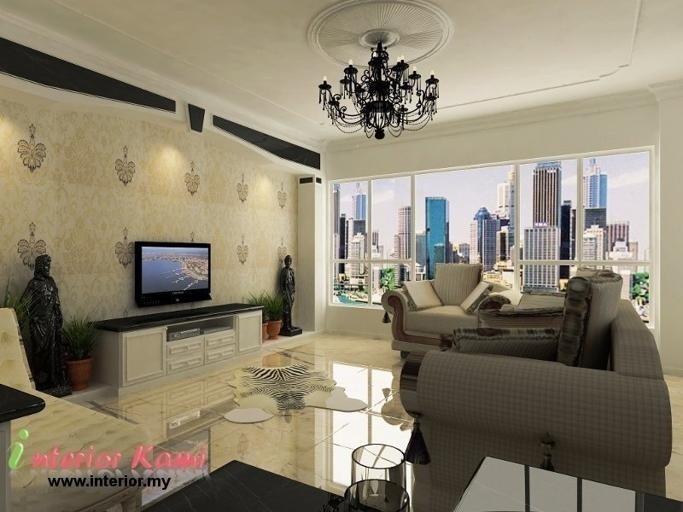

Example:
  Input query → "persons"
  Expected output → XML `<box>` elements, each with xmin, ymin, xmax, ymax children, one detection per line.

<box><xmin>14</xmin><ymin>254</ymin><xmax>73</xmax><ymax>397</ymax></box>
<box><xmin>278</xmin><ymin>254</ymin><xmax>302</xmax><ymax>337</ymax></box>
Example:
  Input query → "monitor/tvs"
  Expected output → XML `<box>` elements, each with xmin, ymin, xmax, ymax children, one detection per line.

<box><xmin>135</xmin><ymin>240</ymin><xmax>212</xmax><ymax>307</ymax></box>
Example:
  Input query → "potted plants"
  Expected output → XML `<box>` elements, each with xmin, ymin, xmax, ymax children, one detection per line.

<box><xmin>60</xmin><ymin>317</ymin><xmax>94</xmax><ymax>392</ymax></box>
<box><xmin>243</xmin><ymin>289</ymin><xmax>284</xmax><ymax>342</ymax></box>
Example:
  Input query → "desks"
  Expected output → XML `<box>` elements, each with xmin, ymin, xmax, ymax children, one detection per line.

<box><xmin>142</xmin><ymin>452</ymin><xmax>682</xmax><ymax>510</ymax></box>
<box><xmin>0</xmin><ymin>382</ymin><xmax>46</xmax><ymax>511</ymax></box>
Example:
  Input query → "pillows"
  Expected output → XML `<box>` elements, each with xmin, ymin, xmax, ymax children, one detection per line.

<box><xmin>446</xmin><ymin>326</ymin><xmax>559</xmax><ymax>361</ymax></box>
<box><xmin>399</xmin><ymin>260</ymin><xmax>492</xmax><ymax>315</ymax></box>
<box><xmin>557</xmin><ymin>268</ymin><xmax>624</xmax><ymax>368</ymax></box>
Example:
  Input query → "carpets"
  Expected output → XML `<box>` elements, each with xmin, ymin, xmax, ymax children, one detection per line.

<box><xmin>221</xmin><ymin>361</ymin><xmax>369</xmax><ymax>426</ymax></box>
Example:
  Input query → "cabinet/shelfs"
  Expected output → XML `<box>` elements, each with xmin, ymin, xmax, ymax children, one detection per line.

<box><xmin>165</xmin><ymin>335</ymin><xmax>204</xmax><ymax>373</ymax></box>
<box><xmin>204</xmin><ymin>331</ymin><xmax>237</xmax><ymax>364</ymax></box>
<box><xmin>233</xmin><ymin>311</ymin><xmax>262</xmax><ymax>354</ymax></box>
<box><xmin>90</xmin><ymin>328</ymin><xmax>164</xmax><ymax>386</ymax></box>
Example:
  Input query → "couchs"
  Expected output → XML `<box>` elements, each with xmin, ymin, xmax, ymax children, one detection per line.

<box><xmin>0</xmin><ymin>305</ymin><xmax>150</xmax><ymax>510</ymax></box>
<box><xmin>398</xmin><ymin>299</ymin><xmax>672</xmax><ymax>502</ymax></box>
<box><xmin>379</xmin><ymin>279</ymin><xmax>565</xmax><ymax>352</ymax></box>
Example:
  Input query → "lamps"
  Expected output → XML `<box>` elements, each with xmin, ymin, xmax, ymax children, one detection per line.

<box><xmin>303</xmin><ymin>2</ymin><xmax>452</xmax><ymax>141</ymax></box>
